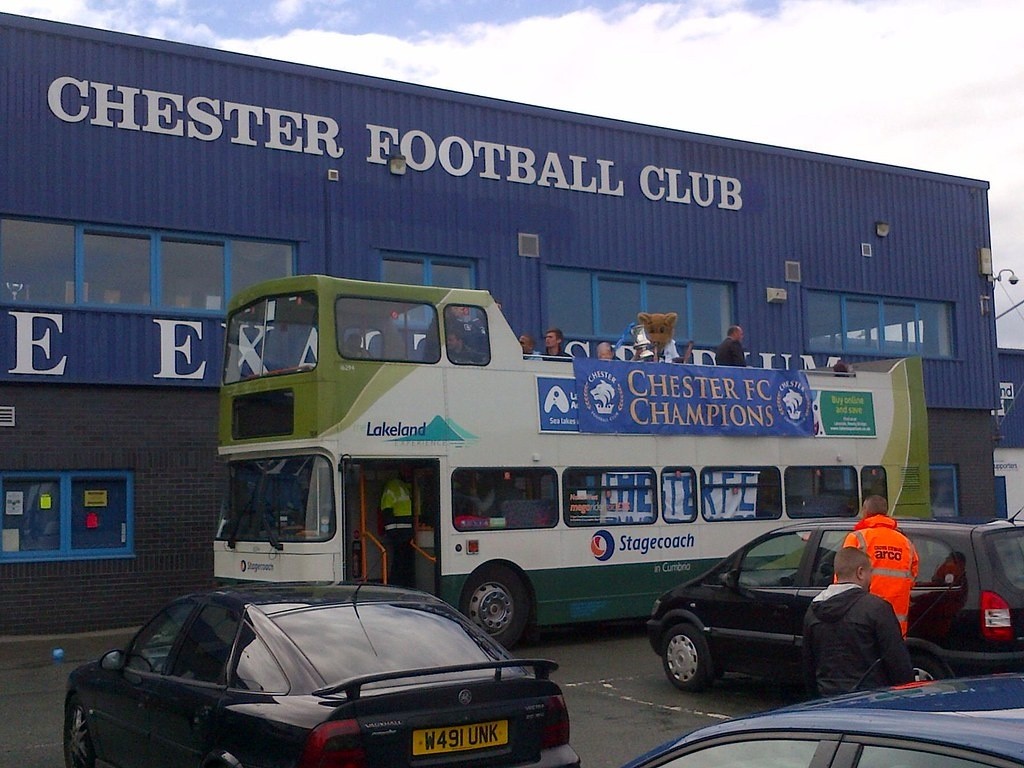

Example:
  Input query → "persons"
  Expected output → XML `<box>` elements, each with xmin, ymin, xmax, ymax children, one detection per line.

<box><xmin>425</xmin><ymin>301</ymin><xmax>504</xmax><ymax>365</ymax></box>
<box><xmin>381</xmin><ymin>464</ymin><xmax>422</xmax><ymax>591</ymax></box>
<box><xmin>342</xmin><ymin>332</ymin><xmax>371</xmax><ymax>358</ymax></box>
<box><xmin>834</xmin><ymin>495</ymin><xmax>918</xmax><ymax>640</ymax></box>
<box><xmin>453</xmin><ymin>470</ymin><xmax>496</xmax><ymax>518</ymax></box>
<box><xmin>714</xmin><ymin>326</ymin><xmax>745</xmax><ymax>367</ymax></box>
<box><xmin>596</xmin><ymin>341</ymin><xmax>621</xmax><ymax>360</ymax></box>
<box><xmin>518</xmin><ymin>335</ymin><xmax>542</xmax><ymax>361</ymax></box>
<box><xmin>801</xmin><ymin>546</ymin><xmax>916</xmax><ymax>699</ymax></box>
<box><xmin>542</xmin><ymin>329</ymin><xmax>572</xmax><ymax>361</ymax></box>
<box><xmin>834</xmin><ymin>364</ymin><xmax>850</xmax><ymax>376</ymax></box>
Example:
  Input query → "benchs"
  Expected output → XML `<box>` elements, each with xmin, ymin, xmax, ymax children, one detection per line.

<box><xmin>761</xmin><ymin>489</ymin><xmax>866</xmax><ymax>520</ymax></box>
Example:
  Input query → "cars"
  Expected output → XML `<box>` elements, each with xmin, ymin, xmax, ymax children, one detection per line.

<box><xmin>62</xmin><ymin>585</ymin><xmax>582</xmax><ymax>768</ymax></box>
<box><xmin>622</xmin><ymin>669</ymin><xmax>1024</xmax><ymax>768</ymax></box>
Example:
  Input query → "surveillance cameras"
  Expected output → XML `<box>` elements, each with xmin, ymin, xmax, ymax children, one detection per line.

<box><xmin>1009</xmin><ymin>276</ymin><xmax>1019</xmax><ymax>284</ymax></box>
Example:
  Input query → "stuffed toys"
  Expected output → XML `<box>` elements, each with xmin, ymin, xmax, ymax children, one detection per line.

<box><xmin>636</xmin><ymin>311</ymin><xmax>679</xmax><ymax>363</ymax></box>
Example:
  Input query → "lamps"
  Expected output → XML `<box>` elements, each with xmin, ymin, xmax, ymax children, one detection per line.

<box><xmin>875</xmin><ymin>220</ymin><xmax>891</xmax><ymax>238</ymax></box>
<box><xmin>388</xmin><ymin>153</ymin><xmax>409</xmax><ymax>175</ymax></box>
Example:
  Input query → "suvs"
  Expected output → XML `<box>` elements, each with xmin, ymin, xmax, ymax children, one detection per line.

<box><xmin>643</xmin><ymin>515</ymin><xmax>1024</xmax><ymax>696</ymax></box>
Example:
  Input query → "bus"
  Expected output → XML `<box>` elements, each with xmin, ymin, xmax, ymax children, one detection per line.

<box><xmin>210</xmin><ymin>274</ymin><xmax>934</xmax><ymax>650</ymax></box>
